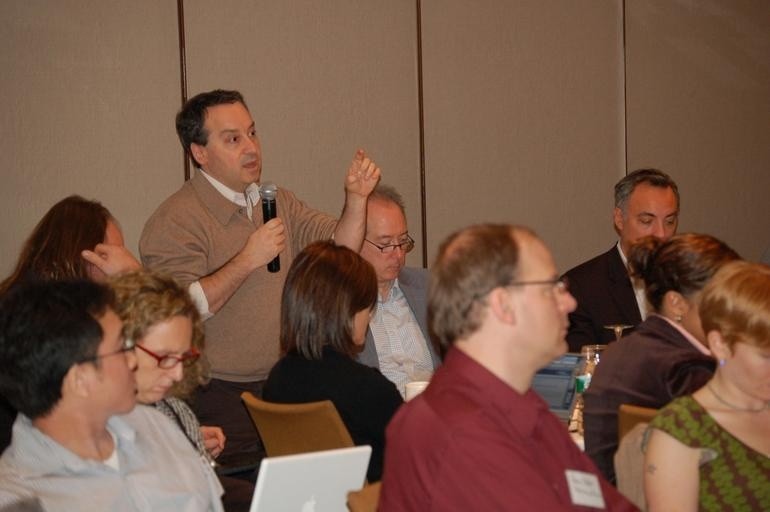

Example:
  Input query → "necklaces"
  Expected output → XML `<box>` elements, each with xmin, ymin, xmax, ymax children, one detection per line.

<box><xmin>703</xmin><ymin>381</ymin><xmax>768</xmax><ymax>413</ymax></box>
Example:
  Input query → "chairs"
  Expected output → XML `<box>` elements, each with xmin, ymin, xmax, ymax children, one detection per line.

<box><xmin>617</xmin><ymin>403</ymin><xmax>659</xmax><ymax>448</ymax></box>
<box><xmin>346</xmin><ymin>479</ymin><xmax>383</xmax><ymax>512</ymax></box>
<box><xmin>241</xmin><ymin>391</ymin><xmax>369</xmax><ymax>491</ymax></box>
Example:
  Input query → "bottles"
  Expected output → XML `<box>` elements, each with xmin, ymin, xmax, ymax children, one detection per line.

<box><xmin>575</xmin><ymin>345</ymin><xmax>597</xmax><ymax>436</ymax></box>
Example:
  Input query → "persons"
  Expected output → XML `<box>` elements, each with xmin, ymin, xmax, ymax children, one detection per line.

<box><xmin>582</xmin><ymin>232</ymin><xmax>746</xmax><ymax>489</ymax></box>
<box><xmin>644</xmin><ymin>260</ymin><xmax>770</xmax><ymax>511</ymax></box>
<box><xmin>106</xmin><ymin>267</ymin><xmax>216</xmax><ymax>469</ymax></box>
<box><xmin>1</xmin><ymin>194</ymin><xmax>227</xmax><ymax>461</ymax></box>
<box><xmin>264</xmin><ymin>237</ymin><xmax>407</xmax><ymax>483</ymax></box>
<box><xmin>0</xmin><ymin>277</ymin><xmax>228</xmax><ymax>510</ymax></box>
<box><xmin>381</xmin><ymin>222</ymin><xmax>643</xmax><ymax>512</ymax></box>
<box><xmin>355</xmin><ymin>185</ymin><xmax>442</xmax><ymax>402</ymax></box>
<box><xmin>137</xmin><ymin>86</ymin><xmax>382</xmax><ymax>511</ymax></box>
<box><xmin>557</xmin><ymin>167</ymin><xmax>681</xmax><ymax>353</ymax></box>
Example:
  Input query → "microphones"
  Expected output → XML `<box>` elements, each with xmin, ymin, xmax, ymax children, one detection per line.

<box><xmin>258</xmin><ymin>180</ymin><xmax>281</xmax><ymax>272</ymax></box>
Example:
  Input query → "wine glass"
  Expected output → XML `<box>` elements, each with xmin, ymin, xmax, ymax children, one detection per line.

<box><xmin>602</xmin><ymin>322</ymin><xmax>634</xmax><ymax>341</ymax></box>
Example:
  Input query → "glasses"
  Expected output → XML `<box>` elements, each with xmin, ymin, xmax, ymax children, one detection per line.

<box><xmin>502</xmin><ymin>276</ymin><xmax>569</xmax><ymax>294</ymax></box>
<box><xmin>364</xmin><ymin>236</ymin><xmax>415</xmax><ymax>254</ymax></box>
<box><xmin>79</xmin><ymin>340</ymin><xmax>136</xmax><ymax>364</ymax></box>
<box><xmin>134</xmin><ymin>344</ymin><xmax>201</xmax><ymax>370</ymax></box>
